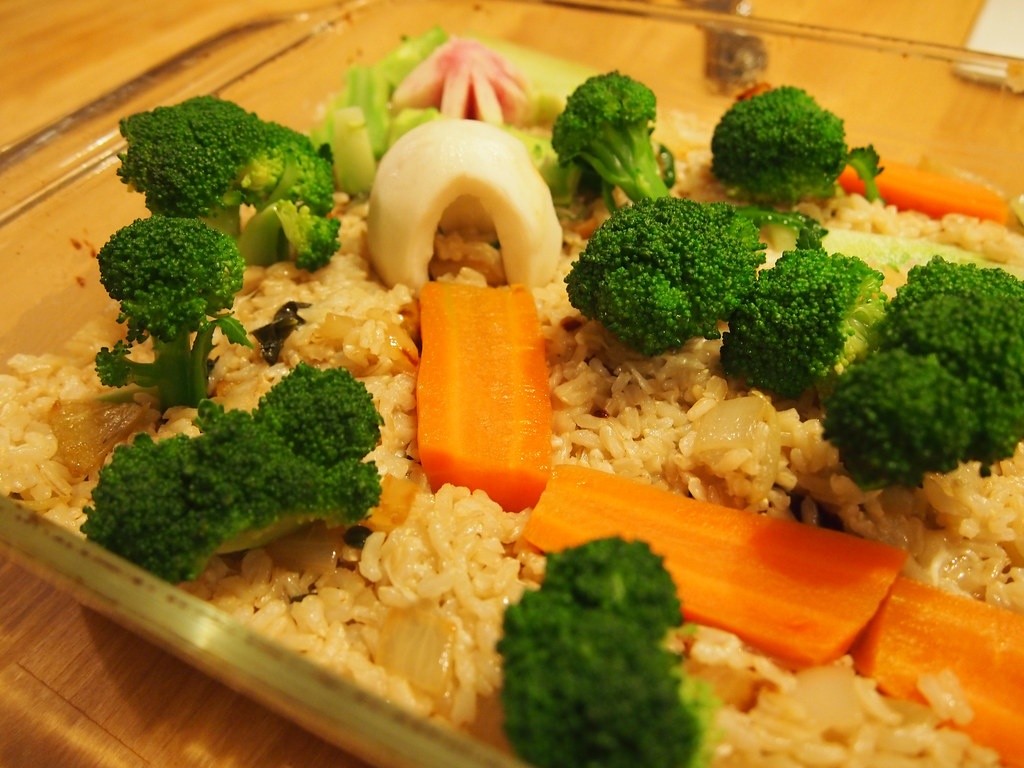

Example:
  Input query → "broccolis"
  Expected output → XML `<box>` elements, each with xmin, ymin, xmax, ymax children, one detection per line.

<box><xmin>78</xmin><ymin>22</ymin><xmax>1024</xmax><ymax>768</ymax></box>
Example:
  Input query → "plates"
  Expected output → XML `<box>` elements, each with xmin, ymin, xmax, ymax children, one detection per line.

<box><xmin>0</xmin><ymin>0</ymin><xmax>1024</xmax><ymax>768</ymax></box>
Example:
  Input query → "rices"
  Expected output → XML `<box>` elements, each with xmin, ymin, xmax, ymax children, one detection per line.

<box><xmin>1</xmin><ymin>156</ymin><xmax>1024</xmax><ymax>768</ymax></box>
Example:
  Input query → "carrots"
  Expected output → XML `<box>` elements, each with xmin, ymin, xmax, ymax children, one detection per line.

<box><xmin>519</xmin><ymin>465</ymin><xmax>911</xmax><ymax>668</ymax></box>
<box><xmin>415</xmin><ymin>281</ymin><xmax>553</xmax><ymax>511</ymax></box>
<box><xmin>852</xmin><ymin>578</ymin><xmax>1024</xmax><ymax>768</ymax></box>
<box><xmin>836</xmin><ymin>156</ymin><xmax>1008</xmax><ymax>226</ymax></box>
